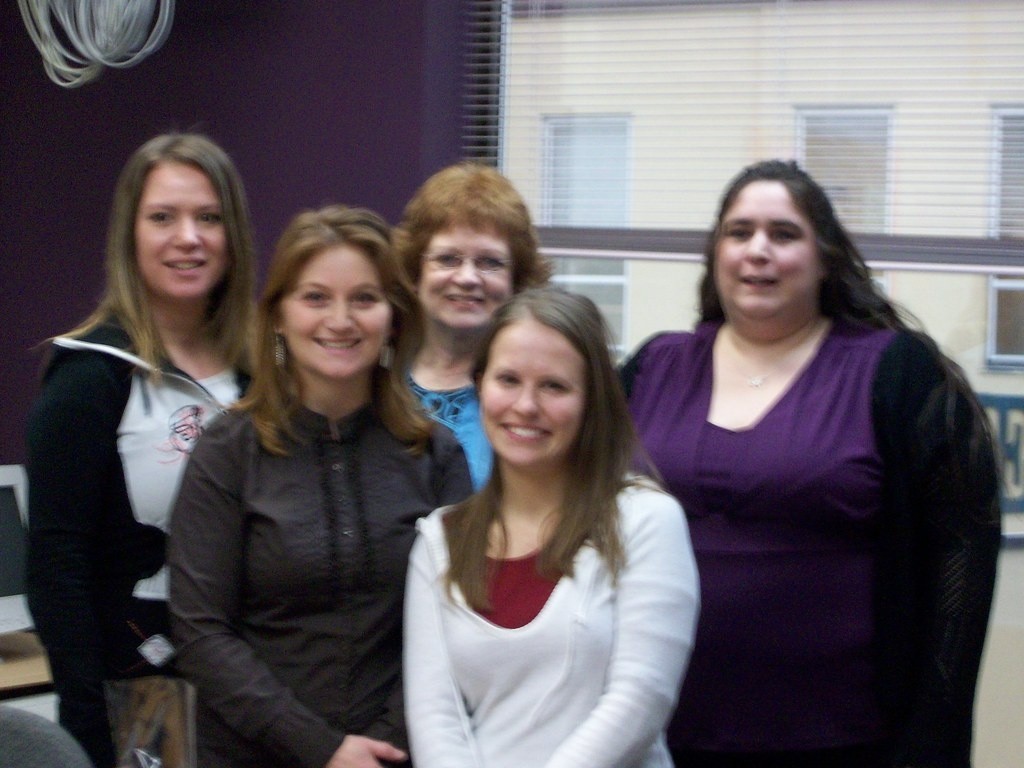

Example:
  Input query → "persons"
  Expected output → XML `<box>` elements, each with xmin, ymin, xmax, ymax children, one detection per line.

<box><xmin>399</xmin><ymin>279</ymin><xmax>702</xmax><ymax>768</ymax></box>
<box><xmin>23</xmin><ymin>130</ymin><xmax>263</xmax><ymax>768</ymax></box>
<box><xmin>165</xmin><ymin>203</ymin><xmax>476</xmax><ymax>768</ymax></box>
<box><xmin>385</xmin><ymin>161</ymin><xmax>559</xmax><ymax>490</ymax></box>
<box><xmin>613</xmin><ymin>157</ymin><xmax>1004</xmax><ymax>768</ymax></box>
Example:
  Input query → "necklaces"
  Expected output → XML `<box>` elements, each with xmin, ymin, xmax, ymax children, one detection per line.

<box><xmin>720</xmin><ymin>323</ymin><xmax>823</xmax><ymax>387</ymax></box>
